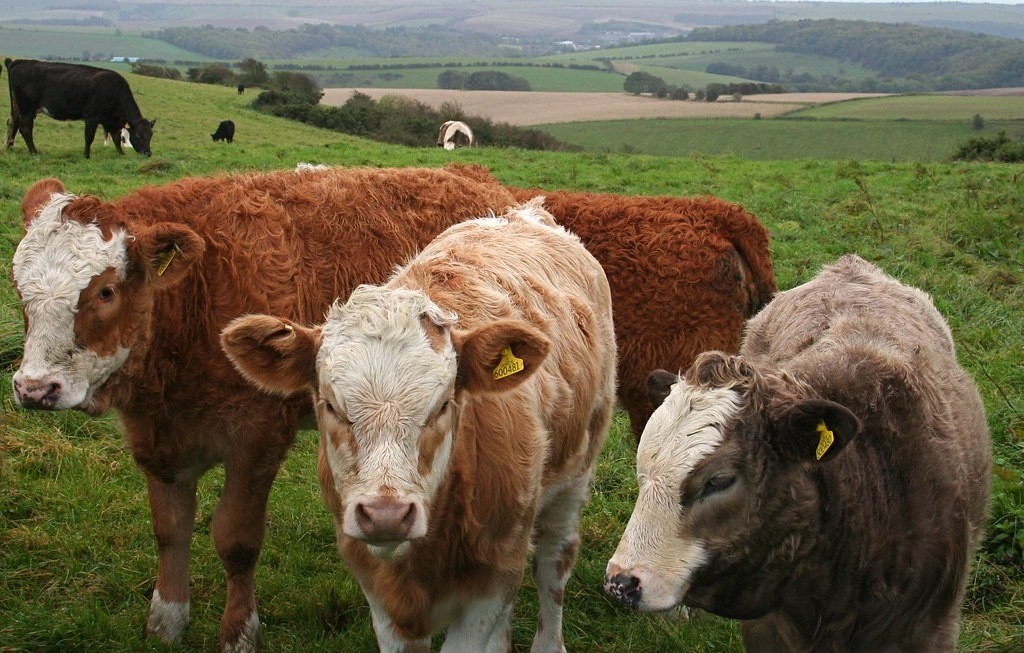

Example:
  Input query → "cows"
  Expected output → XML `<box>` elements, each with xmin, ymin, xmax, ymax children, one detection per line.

<box><xmin>603</xmin><ymin>255</ymin><xmax>990</xmax><ymax>653</ymax></box>
<box><xmin>0</xmin><ymin>58</ymin><xmax>157</xmax><ymax>158</ymax></box>
<box><xmin>219</xmin><ymin>195</ymin><xmax>616</xmax><ymax>653</ymax></box>
<box><xmin>237</xmin><ymin>84</ymin><xmax>245</xmax><ymax>95</ymax></box>
<box><xmin>8</xmin><ymin>159</ymin><xmax>772</xmax><ymax>653</ymax></box>
<box><xmin>437</xmin><ymin>121</ymin><xmax>475</xmax><ymax>151</ymax></box>
<box><xmin>210</xmin><ymin>119</ymin><xmax>236</xmax><ymax>144</ymax></box>
